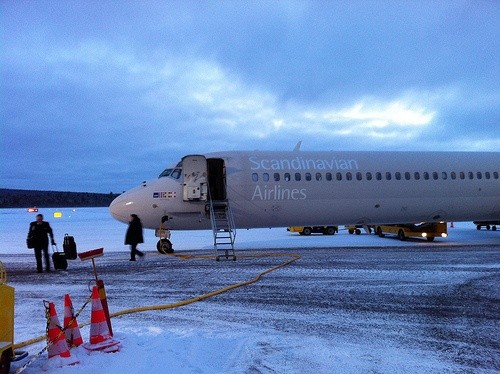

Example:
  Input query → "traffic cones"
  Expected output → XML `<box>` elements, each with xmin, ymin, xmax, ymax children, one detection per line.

<box><xmin>79</xmin><ymin>285</ymin><xmax>121</xmax><ymax>351</ymax></box>
<box><xmin>61</xmin><ymin>294</ymin><xmax>85</xmax><ymax>350</ymax></box>
<box><xmin>41</xmin><ymin>302</ymin><xmax>79</xmax><ymax>368</ymax></box>
<box><xmin>95</xmin><ymin>280</ymin><xmax>116</xmax><ymax>339</ymax></box>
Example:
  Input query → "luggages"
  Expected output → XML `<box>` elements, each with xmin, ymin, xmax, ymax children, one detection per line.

<box><xmin>51</xmin><ymin>243</ymin><xmax>68</xmax><ymax>270</ymax></box>
<box><xmin>63</xmin><ymin>233</ymin><xmax>77</xmax><ymax>260</ymax></box>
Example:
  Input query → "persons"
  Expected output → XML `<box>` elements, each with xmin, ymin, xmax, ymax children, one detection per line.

<box><xmin>125</xmin><ymin>214</ymin><xmax>144</xmax><ymax>261</ymax></box>
<box><xmin>29</xmin><ymin>214</ymin><xmax>56</xmax><ymax>274</ymax></box>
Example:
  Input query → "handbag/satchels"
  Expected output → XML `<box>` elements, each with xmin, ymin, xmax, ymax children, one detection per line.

<box><xmin>64</xmin><ymin>236</ymin><xmax>74</xmax><ymax>244</ymax></box>
<box><xmin>27</xmin><ymin>238</ymin><xmax>34</xmax><ymax>249</ymax></box>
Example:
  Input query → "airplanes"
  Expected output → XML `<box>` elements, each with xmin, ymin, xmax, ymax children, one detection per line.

<box><xmin>108</xmin><ymin>140</ymin><xmax>500</xmax><ymax>263</ymax></box>
